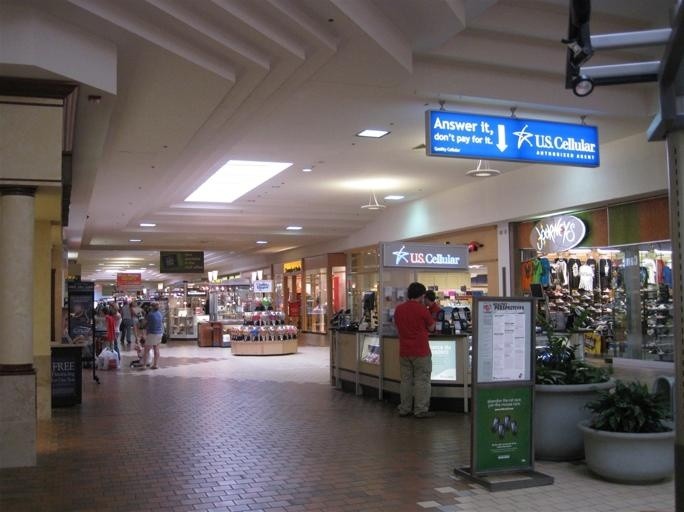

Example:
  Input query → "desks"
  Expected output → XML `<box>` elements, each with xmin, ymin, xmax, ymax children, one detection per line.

<box><xmin>328</xmin><ymin>327</ymin><xmax>472</xmax><ymax>414</ymax></box>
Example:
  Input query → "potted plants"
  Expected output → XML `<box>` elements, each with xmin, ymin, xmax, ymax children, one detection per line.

<box><xmin>532</xmin><ymin>302</ymin><xmax>613</xmax><ymax>461</ymax></box>
<box><xmin>578</xmin><ymin>381</ymin><xmax>674</xmax><ymax>484</ymax></box>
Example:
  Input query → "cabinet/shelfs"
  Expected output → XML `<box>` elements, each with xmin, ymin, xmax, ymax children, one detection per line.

<box><xmin>169</xmin><ymin>286</ymin><xmax>191</xmax><ymax>339</ymax></box>
<box><xmin>209</xmin><ymin>277</ymin><xmax>253</xmax><ymax>347</ymax></box>
<box><xmin>533</xmin><ymin>250</ymin><xmax>613</xmax><ymax>356</ymax></box>
<box><xmin>185</xmin><ymin>281</ymin><xmax>209</xmax><ymax>338</ymax></box>
<box><xmin>151</xmin><ymin>293</ymin><xmax>168</xmax><ymax>340</ymax></box>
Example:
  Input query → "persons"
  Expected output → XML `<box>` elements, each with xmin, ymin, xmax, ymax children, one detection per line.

<box><xmin>423</xmin><ymin>289</ymin><xmax>442</xmax><ymax>321</ymax></box>
<box><xmin>140</xmin><ymin>337</ymin><xmax>152</xmax><ymax>367</ymax></box>
<box><xmin>102</xmin><ymin>302</ymin><xmax>106</xmax><ymax>308</ymax></box>
<box><xmin>394</xmin><ymin>282</ymin><xmax>436</xmax><ymax>419</ymax></box>
<box><xmin>119</xmin><ymin>306</ymin><xmax>122</xmax><ymax>315</ymax></box>
<box><xmin>261</xmin><ymin>297</ymin><xmax>273</xmax><ymax>310</ymax></box>
<box><xmin>203</xmin><ymin>299</ymin><xmax>209</xmax><ymax>314</ymax></box>
<box><xmin>141</xmin><ymin>302</ymin><xmax>152</xmax><ymax>317</ymax></box>
<box><xmin>100</xmin><ymin>307</ymin><xmax>116</xmax><ymax>352</ymax></box>
<box><xmin>138</xmin><ymin>302</ymin><xmax>163</xmax><ymax>370</ymax></box>
<box><xmin>106</xmin><ymin>302</ymin><xmax>109</xmax><ymax>308</ymax></box>
<box><xmin>254</xmin><ymin>298</ymin><xmax>265</xmax><ymax>311</ymax></box>
<box><xmin>313</xmin><ymin>296</ymin><xmax>327</xmax><ymax>313</ymax></box>
<box><xmin>119</xmin><ymin>301</ymin><xmax>133</xmax><ymax>345</ymax></box>
<box><xmin>130</xmin><ymin>301</ymin><xmax>143</xmax><ymax>345</ymax></box>
<box><xmin>111</xmin><ymin>303</ymin><xmax>122</xmax><ymax>364</ymax></box>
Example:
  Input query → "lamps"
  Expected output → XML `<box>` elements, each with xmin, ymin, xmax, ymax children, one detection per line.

<box><xmin>466</xmin><ymin>161</ymin><xmax>501</xmax><ymax>178</ymax></box>
<box><xmin>184</xmin><ymin>160</ymin><xmax>293</xmax><ymax>203</ymax></box>
<box><xmin>574</xmin><ymin>68</ymin><xmax>594</xmax><ymax>97</ymax></box>
<box><xmin>361</xmin><ymin>191</ymin><xmax>386</xmax><ymax>210</ymax></box>
